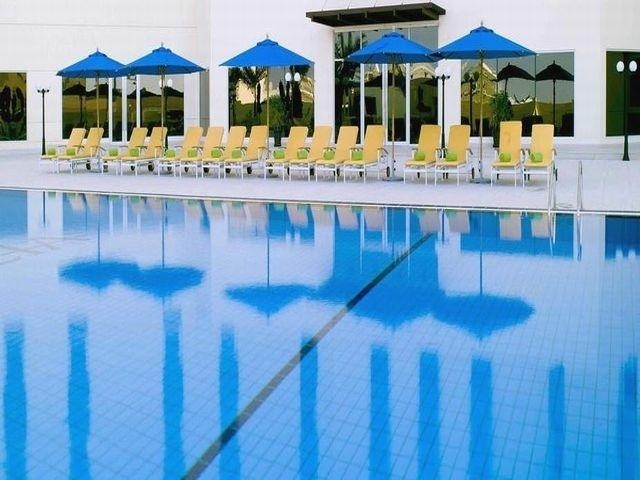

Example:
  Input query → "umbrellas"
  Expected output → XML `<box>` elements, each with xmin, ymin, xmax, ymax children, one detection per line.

<box><xmin>490</xmin><ymin>59</ymin><xmax>574</xmax><ymax>125</ymax></box>
<box><xmin>218</xmin><ymin>34</ymin><xmax>315</xmax><ymax>173</ymax></box>
<box><xmin>346</xmin><ymin>27</ymin><xmax>442</xmax><ymax>178</ymax></box>
<box><xmin>116</xmin><ymin>42</ymin><xmax>208</xmax><ymax>171</ymax></box>
<box><xmin>55</xmin><ymin>48</ymin><xmax>128</xmax><ymax>127</ymax></box>
<box><xmin>430</xmin><ymin>21</ymin><xmax>537</xmax><ymax>178</ymax></box>
<box><xmin>63</xmin><ymin>82</ymin><xmax>183</xmax><ymax>127</ymax></box>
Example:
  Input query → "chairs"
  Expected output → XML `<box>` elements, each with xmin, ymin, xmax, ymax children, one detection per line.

<box><xmin>179</xmin><ymin>127</ymin><xmax>224</xmax><ymax>179</ymax></box>
<box><xmin>315</xmin><ymin>127</ymin><xmax>359</xmax><ymax>183</ymax></box>
<box><xmin>156</xmin><ymin>127</ymin><xmax>203</xmax><ymax>178</ymax></box>
<box><xmin>55</xmin><ymin>128</ymin><xmax>106</xmax><ymax>176</ymax></box>
<box><xmin>64</xmin><ymin>192</ymin><xmax>560</xmax><ymax>245</ymax></box>
<box><xmin>120</xmin><ymin>127</ymin><xmax>168</xmax><ymax>177</ymax></box>
<box><xmin>489</xmin><ymin>121</ymin><xmax>525</xmax><ymax>188</ymax></box>
<box><xmin>221</xmin><ymin>126</ymin><xmax>269</xmax><ymax>181</ymax></box>
<box><xmin>100</xmin><ymin>128</ymin><xmax>148</xmax><ymax>177</ymax></box>
<box><xmin>41</xmin><ymin>128</ymin><xmax>87</xmax><ymax>174</ymax></box>
<box><xmin>201</xmin><ymin>126</ymin><xmax>247</xmax><ymax>180</ymax></box>
<box><xmin>523</xmin><ymin>123</ymin><xmax>558</xmax><ymax>188</ymax></box>
<box><xmin>262</xmin><ymin>126</ymin><xmax>308</xmax><ymax>181</ymax></box>
<box><xmin>401</xmin><ymin>124</ymin><xmax>442</xmax><ymax>185</ymax></box>
<box><xmin>288</xmin><ymin>126</ymin><xmax>333</xmax><ymax>181</ymax></box>
<box><xmin>342</xmin><ymin>125</ymin><xmax>396</xmax><ymax>184</ymax></box>
<box><xmin>434</xmin><ymin>124</ymin><xmax>475</xmax><ymax>187</ymax></box>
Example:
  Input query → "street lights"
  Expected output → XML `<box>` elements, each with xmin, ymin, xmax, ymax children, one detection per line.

<box><xmin>616</xmin><ymin>60</ymin><xmax>639</xmax><ymax>160</ymax></box>
<box><xmin>285</xmin><ymin>71</ymin><xmax>303</xmax><ymax>127</ymax></box>
<box><xmin>37</xmin><ymin>88</ymin><xmax>50</xmax><ymax>156</ymax></box>
<box><xmin>157</xmin><ymin>79</ymin><xmax>174</xmax><ymax>129</ymax></box>
<box><xmin>463</xmin><ymin>72</ymin><xmax>479</xmax><ymax>132</ymax></box>
